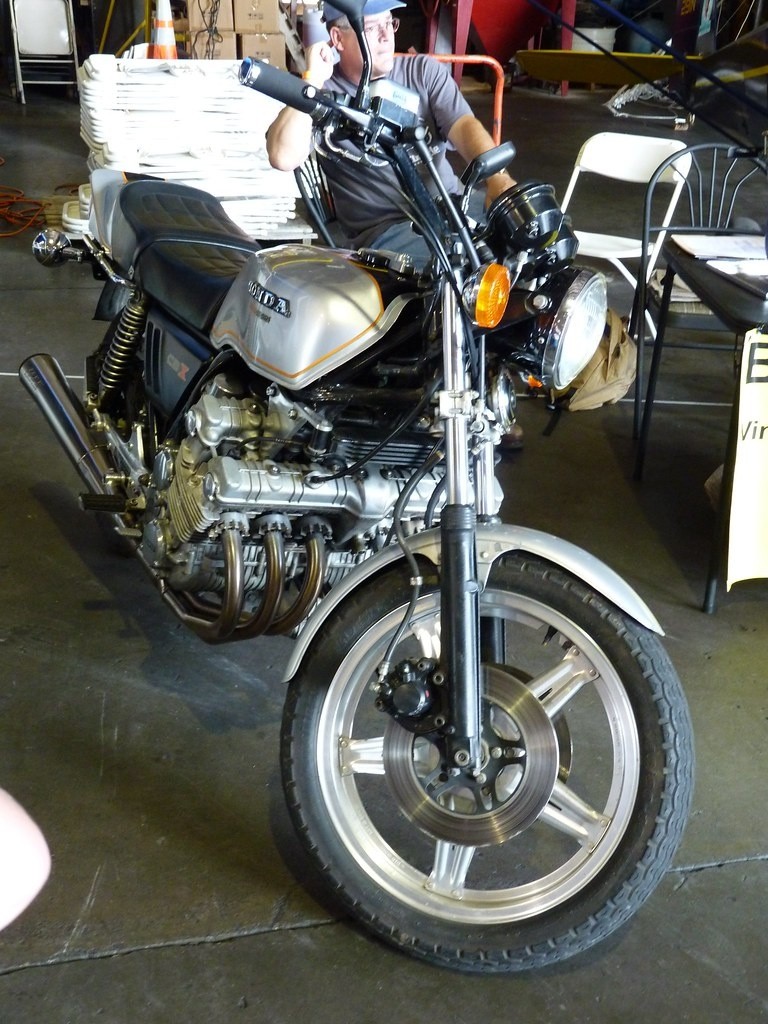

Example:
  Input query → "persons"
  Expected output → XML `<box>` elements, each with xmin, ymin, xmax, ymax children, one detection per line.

<box><xmin>265</xmin><ymin>0</ymin><xmax>525</xmax><ymax>450</ymax></box>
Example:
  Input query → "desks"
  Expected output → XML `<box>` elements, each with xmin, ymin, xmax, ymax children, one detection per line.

<box><xmin>635</xmin><ymin>235</ymin><xmax>768</xmax><ymax>615</ymax></box>
<box><xmin>627</xmin><ymin>142</ymin><xmax>768</xmax><ymax>473</ymax></box>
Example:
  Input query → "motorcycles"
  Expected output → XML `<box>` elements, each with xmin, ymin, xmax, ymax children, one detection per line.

<box><xmin>18</xmin><ymin>0</ymin><xmax>696</xmax><ymax>980</ymax></box>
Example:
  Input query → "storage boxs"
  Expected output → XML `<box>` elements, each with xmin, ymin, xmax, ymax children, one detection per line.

<box><xmin>188</xmin><ymin>0</ymin><xmax>290</xmax><ymax>73</ymax></box>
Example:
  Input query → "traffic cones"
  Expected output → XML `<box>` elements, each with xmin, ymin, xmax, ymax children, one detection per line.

<box><xmin>153</xmin><ymin>0</ymin><xmax>179</xmax><ymax>59</ymax></box>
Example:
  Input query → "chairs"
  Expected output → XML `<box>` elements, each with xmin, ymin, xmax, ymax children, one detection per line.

<box><xmin>298</xmin><ymin>153</ymin><xmax>340</xmax><ymax>249</ymax></box>
<box><xmin>560</xmin><ymin>131</ymin><xmax>692</xmax><ymax>337</ymax></box>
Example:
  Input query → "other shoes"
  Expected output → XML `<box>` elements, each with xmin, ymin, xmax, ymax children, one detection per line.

<box><xmin>501</xmin><ymin>424</ymin><xmax>524</xmax><ymax>450</ymax></box>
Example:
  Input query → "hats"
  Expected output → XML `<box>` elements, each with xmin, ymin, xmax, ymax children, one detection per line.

<box><xmin>320</xmin><ymin>0</ymin><xmax>407</xmax><ymax>23</ymax></box>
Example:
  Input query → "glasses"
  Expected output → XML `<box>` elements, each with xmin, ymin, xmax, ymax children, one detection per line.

<box><xmin>337</xmin><ymin>18</ymin><xmax>400</xmax><ymax>33</ymax></box>
